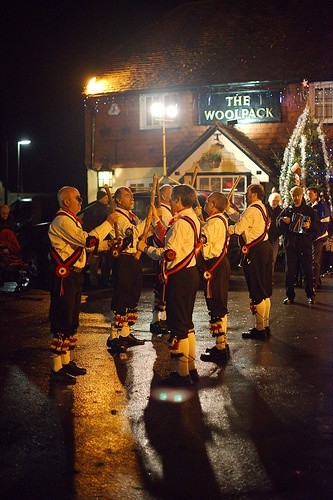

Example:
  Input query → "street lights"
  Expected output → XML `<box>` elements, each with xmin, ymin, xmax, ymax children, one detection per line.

<box><xmin>16</xmin><ymin>139</ymin><xmax>32</xmax><ymax>199</ymax></box>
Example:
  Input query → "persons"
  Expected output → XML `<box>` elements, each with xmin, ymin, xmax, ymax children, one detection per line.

<box><xmin>276</xmin><ymin>186</ymin><xmax>321</xmax><ymax>304</ymax></box>
<box><xmin>108</xmin><ymin>187</ymin><xmax>163</xmax><ymax>353</ymax></box>
<box><xmin>265</xmin><ymin>192</ymin><xmax>282</xmax><ymax>285</ymax></box>
<box><xmin>48</xmin><ymin>186</ymin><xmax>119</xmax><ymax>385</ymax></box>
<box><xmin>149</xmin><ymin>184</ymin><xmax>202</xmax><ymax>334</ymax></box>
<box><xmin>136</xmin><ymin>184</ymin><xmax>200</xmax><ymax>386</ymax></box>
<box><xmin>82</xmin><ymin>190</ymin><xmax>112</xmax><ymax>292</ymax></box>
<box><xmin>224</xmin><ymin>184</ymin><xmax>272</xmax><ymax>340</ymax></box>
<box><xmin>200</xmin><ymin>191</ymin><xmax>231</xmax><ymax>364</ymax></box>
<box><xmin>0</xmin><ymin>205</ymin><xmax>16</xmax><ymax>233</ymax></box>
<box><xmin>294</xmin><ymin>187</ymin><xmax>333</xmax><ymax>292</ymax></box>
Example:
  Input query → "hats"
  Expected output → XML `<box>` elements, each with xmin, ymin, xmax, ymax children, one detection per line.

<box><xmin>97</xmin><ymin>191</ymin><xmax>107</xmax><ymax>201</ymax></box>
<box><xmin>289</xmin><ymin>187</ymin><xmax>303</xmax><ymax>196</ymax></box>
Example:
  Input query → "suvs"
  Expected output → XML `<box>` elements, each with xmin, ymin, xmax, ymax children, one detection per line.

<box><xmin>76</xmin><ymin>191</ymin><xmax>247</xmax><ymax>274</ymax></box>
<box><xmin>4</xmin><ymin>197</ymin><xmax>58</xmax><ymax>279</ymax></box>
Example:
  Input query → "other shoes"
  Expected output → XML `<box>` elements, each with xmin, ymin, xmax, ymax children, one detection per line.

<box><xmin>242</xmin><ymin>328</ymin><xmax>266</xmax><ymax>340</ymax></box>
<box><xmin>106</xmin><ymin>337</ymin><xmax>128</xmax><ymax>350</ymax></box>
<box><xmin>308</xmin><ymin>298</ymin><xmax>313</xmax><ymax>304</ymax></box>
<box><xmin>283</xmin><ymin>297</ymin><xmax>294</xmax><ymax>304</ymax></box>
<box><xmin>161</xmin><ymin>371</ymin><xmax>191</xmax><ymax>387</ymax></box>
<box><xmin>205</xmin><ymin>344</ymin><xmax>230</xmax><ymax>354</ymax></box>
<box><xmin>190</xmin><ymin>368</ymin><xmax>200</xmax><ymax>385</ymax></box>
<box><xmin>63</xmin><ymin>361</ymin><xmax>86</xmax><ymax>376</ymax></box>
<box><xmin>150</xmin><ymin>321</ymin><xmax>166</xmax><ymax>335</ymax></box>
<box><xmin>163</xmin><ymin>319</ymin><xmax>170</xmax><ymax>330</ymax></box>
<box><xmin>249</xmin><ymin>326</ymin><xmax>271</xmax><ymax>335</ymax></box>
<box><xmin>119</xmin><ymin>333</ymin><xmax>144</xmax><ymax>345</ymax></box>
<box><xmin>50</xmin><ymin>367</ymin><xmax>77</xmax><ymax>384</ymax></box>
<box><xmin>200</xmin><ymin>348</ymin><xmax>228</xmax><ymax>363</ymax></box>
